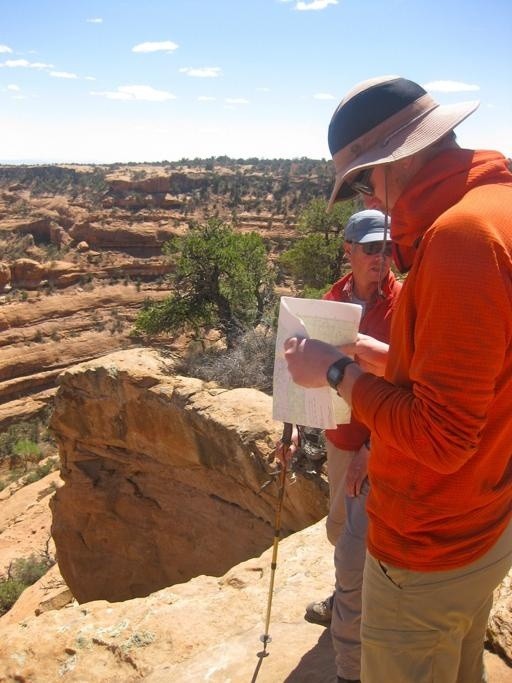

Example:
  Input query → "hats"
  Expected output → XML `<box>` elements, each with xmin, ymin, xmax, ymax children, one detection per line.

<box><xmin>345</xmin><ymin>209</ymin><xmax>392</xmax><ymax>243</ymax></box>
<box><xmin>325</xmin><ymin>77</ymin><xmax>481</xmax><ymax>214</ymax></box>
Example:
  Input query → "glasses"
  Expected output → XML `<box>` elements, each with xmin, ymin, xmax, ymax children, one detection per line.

<box><xmin>363</xmin><ymin>242</ymin><xmax>392</xmax><ymax>257</ymax></box>
<box><xmin>349</xmin><ymin>168</ymin><xmax>374</xmax><ymax>197</ymax></box>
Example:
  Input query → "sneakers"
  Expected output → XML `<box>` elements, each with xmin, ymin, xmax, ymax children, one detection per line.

<box><xmin>306</xmin><ymin>598</ymin><xmax>331</xmax><ymax>623</ymax></box>
